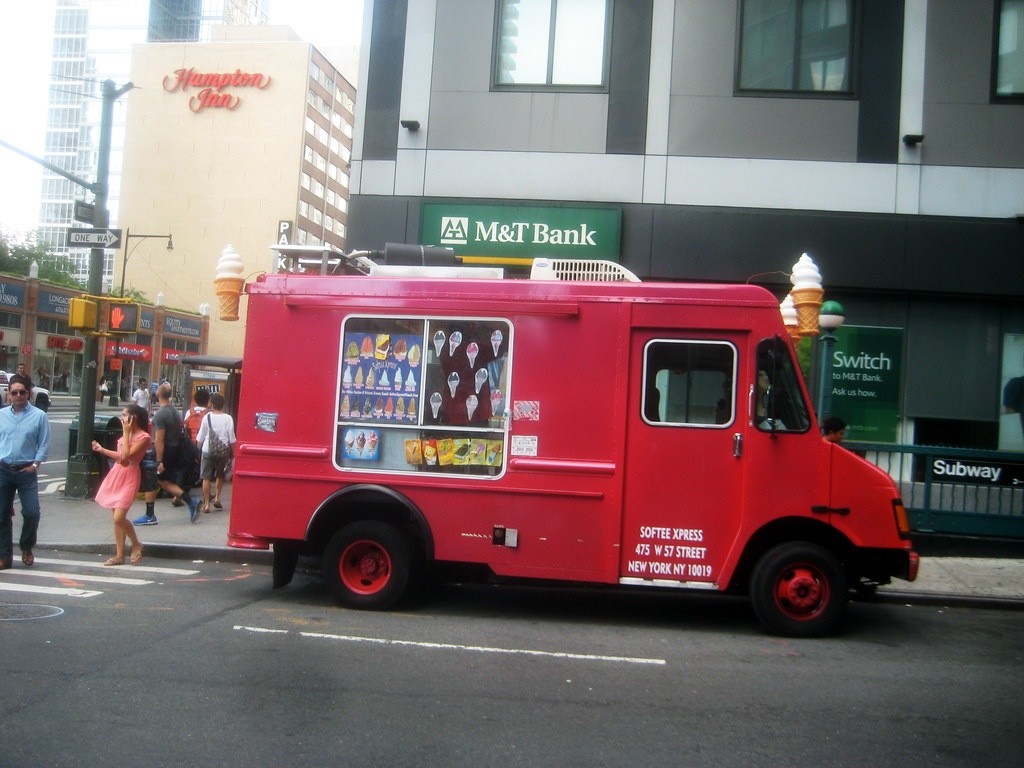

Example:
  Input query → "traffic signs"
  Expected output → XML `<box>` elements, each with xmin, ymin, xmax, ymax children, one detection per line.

<box><xmin>65</xmin><ymin>227</ymin><xmax>122</xmax><ymax>250</ymax></box>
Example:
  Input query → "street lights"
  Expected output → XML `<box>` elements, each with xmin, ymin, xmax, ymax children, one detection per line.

<box><xmin>109</xmin><ymin>227</ymin><xmax>174</xmax><ymax>406</ymax></box>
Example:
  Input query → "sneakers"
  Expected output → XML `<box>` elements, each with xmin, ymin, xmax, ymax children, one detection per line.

<box><xmin>190</xmin><ymin>497</ymin><xmax>202</xmax><ymax>523</ymax></box>
<box><xmin>132</xmin><ymin>514</ymin><xmax>158</xmax><ymax>525</ymax></box>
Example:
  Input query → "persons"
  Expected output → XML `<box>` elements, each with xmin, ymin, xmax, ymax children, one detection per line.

<box><xmin>8</xmin><ymin>364</ymin><xmax>31</xmax><ymax>406</ymax></box>
<box><xmin>0</xmin><ymin>378</ymin><xmax>50</xmax><ymax>570</ymax></box>
<box><xmin>822</xmin><ymin>417</ymin><xmax>847</xmax><ymax>444</ymax></box>
<box><xmin>107</xmin><ymin>376</ymin><xmax>170</xmax><ymax>404</ymax></box>
<box><xmin>91</xmin><ymin>405</ymin><xmax>152</xmax><ymax>566</ymax></box>
<box><xmin>438</xmin><ymin>321</ymin><xmax>508</xmax><ymax>428</ymax></box>
<box><xmin>195</xmin><ymin>393</ymin><xmax>237</xmax><ymax>513</ymax></box>
<box><xmin>132</xmin><ymin>378</ymin><xmax>152</xmax><ymax>422</ymax></box>
<box><xmin>132</xmin><ymin>383</ymin><xmax>202</xmax><ymax>526</ymax></box>
<box><xmin>98</xmin><ymin>375</ymin><xmax>109</xmax><ymax>404</ymax></box>
<box><xmin>171</xmin><ymin>388</ymin><xmax>216</xmax><ymax>506</ymax></box>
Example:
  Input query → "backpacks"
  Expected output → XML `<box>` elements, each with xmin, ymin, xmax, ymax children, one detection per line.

<box><xmin>184</xmin><ymin>406</ymin><xmax>209</xmax><ymax>449</ymax></box>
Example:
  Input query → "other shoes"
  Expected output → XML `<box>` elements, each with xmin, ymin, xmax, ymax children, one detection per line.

<box><xmin>130</xmin><ymin>542</ymin><xmax>144</xmax><ymax>563</ymax></box>
<box><xmin>174</xmin><ymin>499</ymin><xmax>185</xmax><ymax>506</ymax></box>
<box><xmin>104</xmin><ymin>556</ymin><xmax>125</xmax><ymax>565</ymax></box>
<box><xmin>204</xmin><ymin>492</ymin><xmax>216</xmax><ymax>503</ymax></box>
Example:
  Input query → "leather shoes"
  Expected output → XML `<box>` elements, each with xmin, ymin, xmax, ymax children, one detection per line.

<box><xmin>0</xmin><ymin>559</ymin><xmax>12</xmax><ymax>570</ymax></box>
<box><xmin>22</xmin><ymin>548</ymin><xmax>34</xmax><ymax>565</ymax></box>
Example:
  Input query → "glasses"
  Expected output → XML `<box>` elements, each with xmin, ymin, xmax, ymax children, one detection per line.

<box><xmin>10</xmin><ymin>390</ymin><xmax>29</xmax><ymax>396</ymax></box>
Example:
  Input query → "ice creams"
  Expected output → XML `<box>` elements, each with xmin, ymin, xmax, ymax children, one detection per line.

<box><xmin>779</xmin><ymin>292</ymin><xmax>801</xmax><ymax>353</ymax></box>
<box><xmin>343</xmin><ymin>365</ymin><xmax>416</xmax><ymax>392</ymax></box>
<box><xmin>789</xmin><ymin>253</ymin><xmax>824</xmax><ymax>335</ymax></box>
<box><xmin>213</xmin><ymin>243</ymin><xmax>245</xmax><ymax>321</ymax></box>
<box><xmin>345</xmin><ymin>429</ymin><xmax>378</xmax><ymax>450</ymax></box>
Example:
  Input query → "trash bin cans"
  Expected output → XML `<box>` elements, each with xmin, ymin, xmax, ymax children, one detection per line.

<box><xmin>68</xmin><ymin>414</ymin><xmax>123</xmax><ymax>481</ymax></box>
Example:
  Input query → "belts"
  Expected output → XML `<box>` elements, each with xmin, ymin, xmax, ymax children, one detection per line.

<box><xmin>0</xmin><ymin>461</ymin><xmax>34</xmax><ymax>471</ymax></box>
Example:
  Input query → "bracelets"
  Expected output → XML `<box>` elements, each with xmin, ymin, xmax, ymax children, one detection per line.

<box><xmin>155</xmin><ymin>461</ymin><xmax>163</xmax><ymax>470</ymax></box>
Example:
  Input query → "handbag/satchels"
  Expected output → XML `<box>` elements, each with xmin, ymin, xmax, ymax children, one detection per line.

<box><xmin>173</xmin><ymin>459</ymin><xmax>201</xmax><ymax>484</ymax></box>
<box><xmin>207</xmin><ymin>413</ymin><xmax>231</xmax><ymax>458</ymax></box>
<box><xmin>223</xmin><ymin>457</ymin><xmax>235</xmax><ymax>482</ymax></box>
<box><xmin>107</xmin><ymin>453</ymin><xmax>161</xmax><ymax>492</ymax></box>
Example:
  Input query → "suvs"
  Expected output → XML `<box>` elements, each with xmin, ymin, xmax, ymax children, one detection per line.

<box><xmin>0</xmin><ymin>371</ymin><xmax>51</xmax><ymax>413</ymax></box>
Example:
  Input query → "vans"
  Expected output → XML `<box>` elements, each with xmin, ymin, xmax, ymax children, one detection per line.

<box><xmin>227</xmin><ymin>244</ymin><xmax>920</xmax><ymax>637</ymax></box>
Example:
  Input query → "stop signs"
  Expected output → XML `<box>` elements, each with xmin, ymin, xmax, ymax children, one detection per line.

<box><xmin>107</xmin><ymin>301</ymin><xmax>141</xmax><ymax>334</ymax></box>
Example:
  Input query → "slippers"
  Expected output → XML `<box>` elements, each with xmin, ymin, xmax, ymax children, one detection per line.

<box><xmin>213</xmin><ymin>501</ymin><xmax>223</xmax><ymax>508</ymax></box>
<box><xmin>203</xmin><ymin>505</ymin><xmax>210</xmax><ymax>513</ymax></box>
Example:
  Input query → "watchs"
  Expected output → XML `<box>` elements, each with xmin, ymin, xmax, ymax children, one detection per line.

<box><xmin>33</xmin><ymin>463</ymin><xmax>39</xmax><ymax>469</ymax></box>
